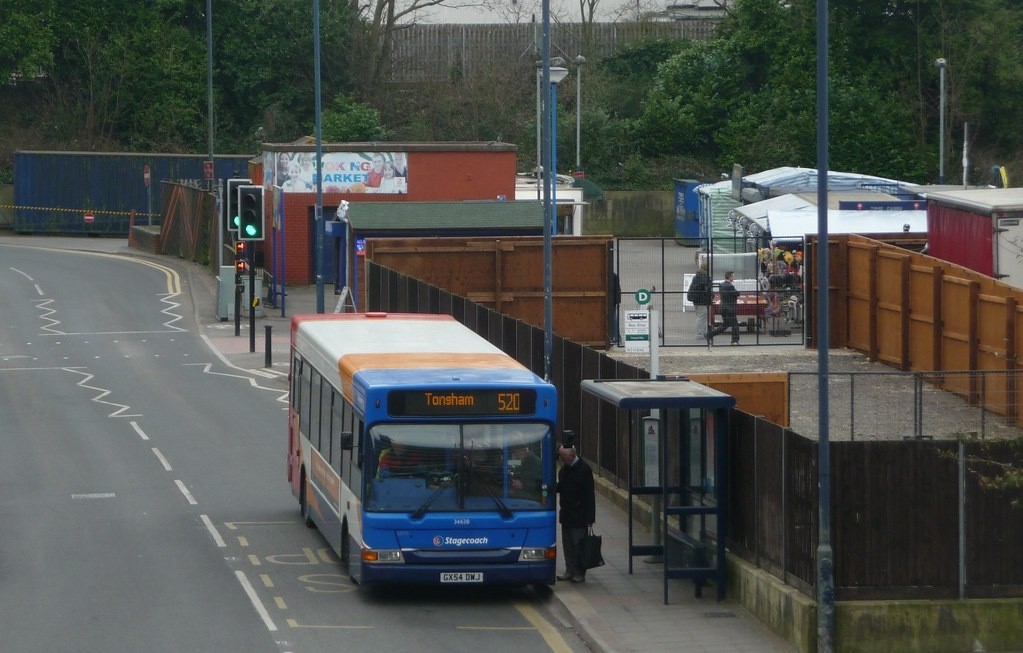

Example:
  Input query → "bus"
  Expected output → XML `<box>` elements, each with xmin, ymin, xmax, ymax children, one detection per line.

<box><xmin>287</xmin><ymin>312</ymin><xmax>561</xmax><ymax>585</ymax></box>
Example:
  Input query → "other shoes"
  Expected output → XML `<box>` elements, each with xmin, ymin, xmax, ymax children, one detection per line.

<box><xmin>572</xmin><ymin>574</ymin><xmax>585</xmax><ymax>583</ymax></box>
<box><xmin>696</xmin><ymin>334</ymin><xmax>705</xmax><ymax>340</ymax></box>
<box><xmin>706</xmin><ymin>334</ymin><xmax>712</xmax><ymax>344</ymax></box>
<box><xmin>557</xmin><ymin>572</ymin><xmax>573</xmax><ymax>580</ymax></box>
<box><xmin>731</xmin><ymin>341</ymin><xmax>737</xmax><ymax>344</ymax></box>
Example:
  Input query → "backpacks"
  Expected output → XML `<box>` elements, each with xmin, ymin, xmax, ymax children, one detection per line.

<box><xmin>687</xmin><ymin>273</ymin><xmax>704</xmax><ymax>301</ymax></box>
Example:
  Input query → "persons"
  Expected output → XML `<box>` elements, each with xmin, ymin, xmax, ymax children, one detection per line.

<box><xmin>363</xmin><ymin>152</ymin><xmax>408</xmax><ymax>193</ymax></box>
<box><xmin>509</xmin><ymin>448</ymin><xmax>542</xmax><ymax>499</ymax></box>
<box><xmin>694</xmin><ymin>264</ymin><xmax>708</xmax><ymax>340</ymax></box>
<box><xmin>706</xmin><ymin>271</ymin><xmax>740</xmax><ymax>345</ymax></box>
<box><xmin>277</xmin><ymin>153</ymin><xmax>313</xmax><ymax>191</ymax></box>
<box><xmin>379</xmin><ymin>437</ymin><xmax>421</xmax><ymax>471</ymax></box>
<box><xmin>455</xmin><ymin>438</ymin><xmax>490</xmax><ymax>478</ymax></box>
<box><xmin>557</xmin><ymin>447</ymin><xmax>596</xmax><ymax>584</ymax></box>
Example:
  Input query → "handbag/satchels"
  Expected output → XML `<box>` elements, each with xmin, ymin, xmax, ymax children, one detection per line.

<box><xmin>579</xmin><ymin>526</ymin><xmax>605</xmax><ymax>569</ymax></box>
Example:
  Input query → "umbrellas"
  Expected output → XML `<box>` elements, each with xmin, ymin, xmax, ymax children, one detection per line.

<box><xmin>573</xmin><ymin>178</ymin><xmax>605</xmax><ymax>200</ymax></box>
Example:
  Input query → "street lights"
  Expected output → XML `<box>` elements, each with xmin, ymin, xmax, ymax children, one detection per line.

<box><xmin>571</xmin><ymin>52</ymin><xmax>587</xmax><ymax>172</ymax></box>
<box><xmin>538</xmin><ymin>65</ymin><xmax>570</xmax><ymax>236</ymax></box>
<box><xmin>933</xmin><ymin>58</ymin><xmax>948</xmax><ymax>185</ymax></box>
<box><xmin>537</xmin><ymin>57</ymin><xmax>566</xmax><ymax>201</ymax></box>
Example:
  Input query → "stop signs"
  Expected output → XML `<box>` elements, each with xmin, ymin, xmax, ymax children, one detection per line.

<box><xmin>84</xmin><ymin>213</ymin><xmax>96</xmax><ymax>225</ymax></box>
<box><xmin>143</xmin><ymin>165</ymin><xmax>151</xmax><ymax>186</ymax></box>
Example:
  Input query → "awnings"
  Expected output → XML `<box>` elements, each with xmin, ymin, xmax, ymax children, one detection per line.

<box><xmin>735</xmin><ymin>194</ymin><xmax>818</xmax><ymax>232</ymax></box>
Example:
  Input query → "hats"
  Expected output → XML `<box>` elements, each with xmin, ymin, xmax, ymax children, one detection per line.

<box><xmin>699</xmin><ymin>261</ymin><xmax>708</xmax><ymax>274</ymax></box>
<box><xmin>758</xmin><ymin>248</ymin><xmax>804</xmax><ymax>323</ymax></box>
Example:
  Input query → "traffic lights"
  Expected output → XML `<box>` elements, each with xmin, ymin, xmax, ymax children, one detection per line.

<box><xmin>234</xmin><ymin>241</ymin><xmax>245</xmax><ymax>256</ymax></box>
<box><xmin>235</xmin><ymin>259</ymin><xmax>245</xmax><ymax>275</ymax></box>
<box><xmin>238</xmin><ymin>185</ymin><xmax>266</xmax><ymax>242</ymax></box>
<box><xmin>226</xmin><ymin>178</ymin><xmax>253</xmax><ymax>231</ymax></box>
